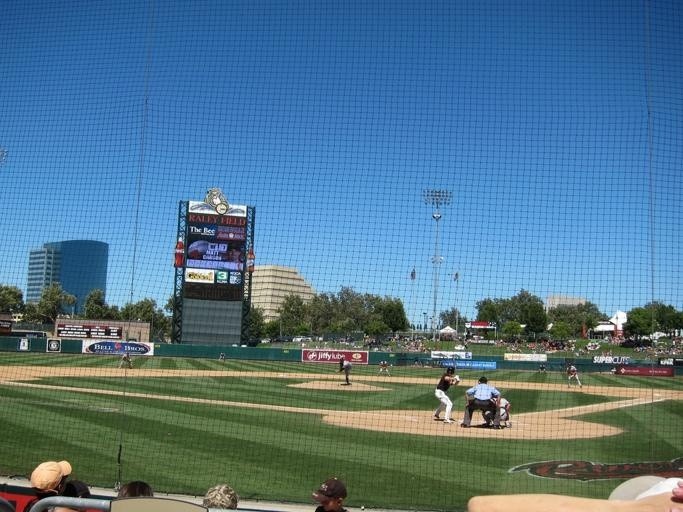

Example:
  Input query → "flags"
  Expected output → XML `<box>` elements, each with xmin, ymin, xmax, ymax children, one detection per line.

<box><xmin>410</xmin><ymin>268</ymin><xmax>416</xmax><ymax>279</ymax></box>
<box><xmin>453</xmin><ymin>272</ymin><xmax>459</xmax><ymax>281</ymax></box>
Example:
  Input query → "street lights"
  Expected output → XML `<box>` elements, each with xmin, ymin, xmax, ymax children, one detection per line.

<box><xmin>423</xmin><ymin>189</ymin><xmax>453</xmax><ymax>329</ymax></box>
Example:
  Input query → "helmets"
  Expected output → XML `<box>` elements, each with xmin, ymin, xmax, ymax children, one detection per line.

<box><xmin>447</xmin><ymin>366</ymin><xmax>454</xmax><ymax>374</ymax></box>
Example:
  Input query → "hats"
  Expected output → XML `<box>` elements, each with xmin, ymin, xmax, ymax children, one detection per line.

<box><xmin>30</xmin><ymin>460</ymin><xmax>71</xmax><ymax>491</ymax></box>
<box><xmin>311</xmin><ymin>479</ymin><xmax>348</xmax><ymax>503</ymax></box>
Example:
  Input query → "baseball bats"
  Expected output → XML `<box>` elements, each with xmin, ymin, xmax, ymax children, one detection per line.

<box><xmin>452</xmin><ymin>358</ymin><xmax>457</xmax><ymax>375</ymax></box>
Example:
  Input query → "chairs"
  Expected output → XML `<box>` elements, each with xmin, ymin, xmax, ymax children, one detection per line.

<box><xmin>109</xmin><ymin>496</ymin><xmax>209</xmax><ymax>512</ymax></box>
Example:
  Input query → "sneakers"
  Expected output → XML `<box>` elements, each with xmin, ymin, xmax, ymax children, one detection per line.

<box><xmin>434</xmin><ymin>414</ymin><xmax>512</xmax><ymax>430</ymax></box>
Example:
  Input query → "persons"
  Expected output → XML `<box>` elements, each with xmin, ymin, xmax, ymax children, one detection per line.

<box><xmin>336</xmin><ymin>357</ymin><xmax>353</xmax><ymax>385</ymax></box>
<box><xmin>310</xmin><ymin>477</ymin><xmax>351</xmax><ymax>512</ymax></box>
<box><xmin>460</xmin><ymin>377</ymin><xmax>502</xmax><ymax>429</ymax></box>
<box><xmin>482</xmin><ymin>394</ymin><xmax>512</xmax><ymax>428</ymax></box>
<box><xmin>668</xmin><ymin>480</ymin><xmax>682</xmax><ymax>512</ymax></box>
<box><xmin>377</xmin><ymin>360</ymin><xmax>391</xmax><ymax>377</ymax></box>
<box><xmin>218</xmin><ymin>352</ymin><xmax>226</xmax><ymax>360</ymax></box>
<box><xmin>55</xmin><ymin>480</ymin><xmax>91</xmax><ymax>512</ymax></box>
<box><xmin>493</xmin><ymin>335</ymin><xmax>683</xmax><ymax>357</ymax></box>
<box><xmin>331</xmin><ymin>333</ymin><xmax>430</xmax><ymax>353</ymax></box>
<box><xmin>565</xmin><ymin>362</ymin><xmax>583</xmax><ymax>388</ymax></box>
<box><xmin>116</xmin><ymin>480</ymin><xmax>153</xmax><ymax>498</ymax></box>
<box><xmin>538</xmin><ymin>361</ymin><xmax>547</xmax><ymax>375</ymax></box>
<box><xmin>118</xmin><ymin>352</ymin><xmax>133</xmax><ymax>369</ymax></box>
<box><xmin>20</xmin><ymin>459</ymin><xmax>73</xmax><ymax>512</ymax></box>
<box><xmin>433</xmin><ymin>366</ymin><xmax>461</xmax><ymax>423</ymax></box>
